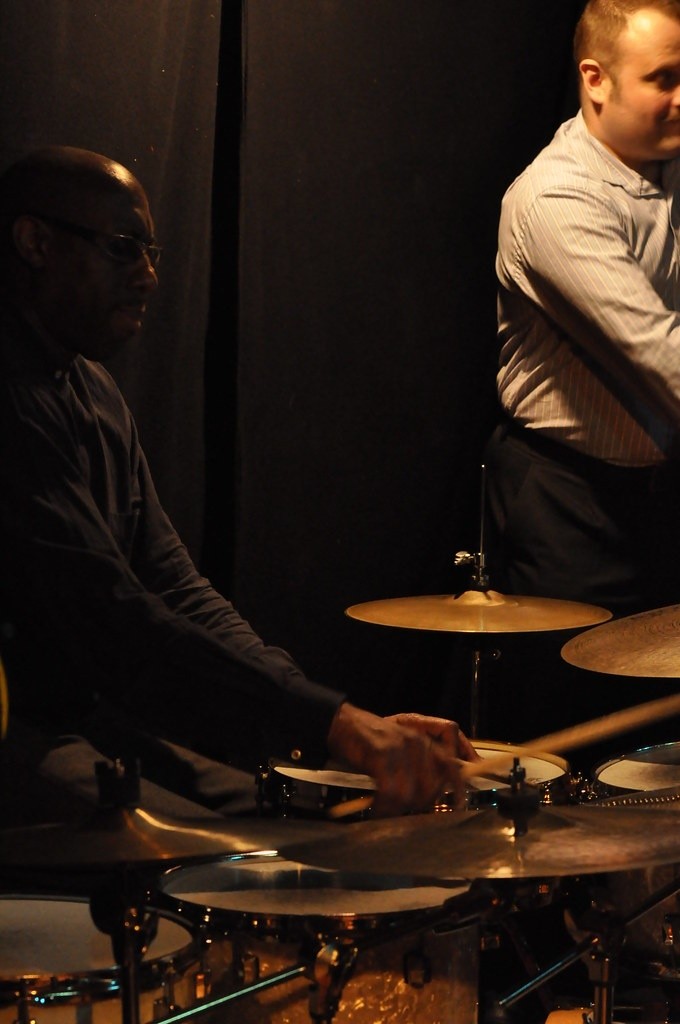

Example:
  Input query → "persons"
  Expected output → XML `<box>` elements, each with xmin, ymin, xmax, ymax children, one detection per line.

<box><xmin>478</xmin><ymin>0</ymin><xmax>680</xmax><ymax>774</ymax></box>
<box><xmin>0</xmin><ymin>144</ymin><xmax>479</xmax><ymax>822</ymax></box>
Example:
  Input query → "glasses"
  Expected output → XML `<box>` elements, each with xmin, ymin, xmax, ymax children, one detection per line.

<box><xmin>17</xmin><ymin>207</ymin><xmax>165</xmax><ymax>277</ymax></box>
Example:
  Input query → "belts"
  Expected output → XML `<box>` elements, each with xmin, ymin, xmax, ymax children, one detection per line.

<box><xmin>506</xmin><ymin>419</ymin><xmax>680</xmax><ymax>493</ymax></box>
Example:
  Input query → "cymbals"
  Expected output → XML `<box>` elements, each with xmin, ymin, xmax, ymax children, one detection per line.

<box><xmin>0</xmin><ymin>810</ymin><xmax>313</xmax><ymax>871</ymax></box>
<box><xmin>345</xmin><ymin>591</ymin><xmax>613</xmax><ymax>633</ymax></box>
<box><xmin>275</xmin><ymin>803</ymin><xmax>679</xmax><ymax>880</ymax></box>
<box><xmin>559</xmin><ymin>603</ymin><xmax>680</xmax><ymax>682</ymax></box>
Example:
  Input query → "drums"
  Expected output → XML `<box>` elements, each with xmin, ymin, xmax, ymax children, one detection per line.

<box><xmin>256</xmin><ymin>737</ymin><xmax>575</xmax><ymax>950</ymax></box>
<box><xmin>589</xmin><ymin>738</ymin><xmax>680</xmax><ymax>984</ymax></box>
<box><xmin>153</xmin><ymin>848</ymin><xmax>483</xmax><ymax>1024</ymax></box>
<box><xmin>1</xmin><ymin>894</ymin><xmax>210</xmax><ymax>1024</ymax></box>
